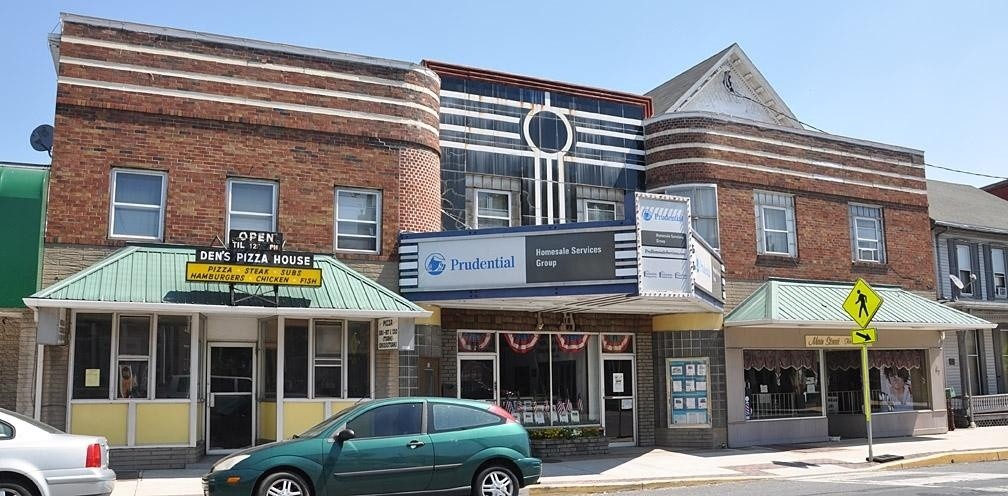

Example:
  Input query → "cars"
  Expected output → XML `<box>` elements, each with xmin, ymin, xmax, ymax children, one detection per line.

<box><xmin>202</xmin><ymin>395</ymin><xmax>542</xmax><ymax>496</ymax></box>
<box><xmin>0</xmin><ymin>406</ymin><xmax>117</xmax><ymax>496</ymax></box>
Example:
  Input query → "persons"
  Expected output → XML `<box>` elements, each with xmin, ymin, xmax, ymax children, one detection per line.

<box><xmin>884</xmin><ymin>367</ymin><xmax>911</xmax><ymax>405</ymax></box>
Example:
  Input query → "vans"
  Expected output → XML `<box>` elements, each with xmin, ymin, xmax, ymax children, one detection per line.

<box><xmin>161</xmin><ymin>373</ymin><xmax>252</xmax><ymax>399</ymax></box>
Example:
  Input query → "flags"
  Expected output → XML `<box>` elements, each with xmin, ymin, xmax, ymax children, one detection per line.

<box><xmin>502</xmin><ymin>392</ymin><xmax>584</xmax><ymax>416</ymax></box>
